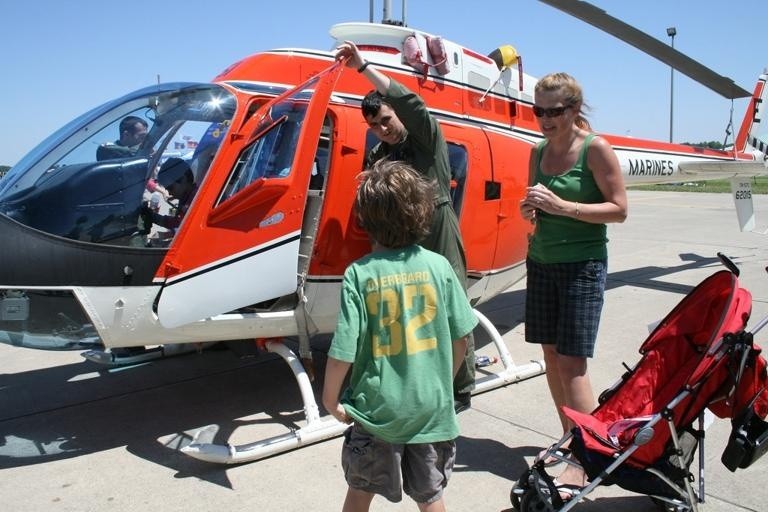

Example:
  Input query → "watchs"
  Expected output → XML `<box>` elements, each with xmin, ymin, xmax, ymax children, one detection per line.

<box><xmin>357</xmin><ymin>59</ymin><xmax>369</xmax><ymax>73</ymax></box>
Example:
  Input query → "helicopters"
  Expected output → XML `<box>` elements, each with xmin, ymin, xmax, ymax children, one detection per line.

<box><xmin>0</xmin><ymin>2</ymin><xmax>767</xmax><ymax>464</ymax></box>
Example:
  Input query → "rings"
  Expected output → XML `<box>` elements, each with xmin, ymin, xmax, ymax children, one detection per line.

<box><xmin>536</xmin><ymin>199</ymin><xmax>541</xmax><ymax>204</ymax></box>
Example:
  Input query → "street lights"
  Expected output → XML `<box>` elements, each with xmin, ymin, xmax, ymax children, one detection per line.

<box><xmin>665</xmin><ymin>26</ymin><xmax>678</xmax><ymax>142</ymax></box>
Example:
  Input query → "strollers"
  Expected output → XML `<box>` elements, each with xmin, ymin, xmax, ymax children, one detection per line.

<box><xmin>508</xmin><ymin>248</ymin><xmax>768</xmax><ymax>512</ymax></box>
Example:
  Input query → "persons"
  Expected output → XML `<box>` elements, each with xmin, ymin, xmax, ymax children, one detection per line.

<box><xmin>335</xmin><ymin>41</ymin><xmax>475</xmax><ymax>413</ymax></box>
<box><xmin>97</xmin><ymin>117</ymin><xmax>199</xmax><ymax>228</ymax></box>
<box><xmin>321</xmin><ymin>156</ymin><xmax>479</xmax><ymax>512</ymax></box>
<box><xmin>519</xmin><ymin>73</ymin><xmax>628</xmax><ymax>501</ymax></box>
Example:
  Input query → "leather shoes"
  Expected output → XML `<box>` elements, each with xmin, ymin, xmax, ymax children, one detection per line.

<box><xmin>453</xmin><ymin>393</ymin><xmax>470</xmax><ymax>414</ymax></box>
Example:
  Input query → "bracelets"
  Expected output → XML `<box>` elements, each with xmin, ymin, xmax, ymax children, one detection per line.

<box><xmin>575</xmin><ymin>201</ymin><xmax>579</xmax><ymax>220</ymax></box>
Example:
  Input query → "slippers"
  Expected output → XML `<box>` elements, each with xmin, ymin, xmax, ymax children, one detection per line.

<box><xmin>533</xmin><ymin>443</ymin><xmax>572</xmax><ymax>467</ymax></box>
<box><xmin>551</xmin><ymin>476</ymin><xmax>586</xmax><ymax>501</ymax></box>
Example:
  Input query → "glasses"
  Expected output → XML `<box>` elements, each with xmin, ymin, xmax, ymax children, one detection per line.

<box><xmin>531</xmin><ymin>105</ymin><xmax>566</xmax><ymax>118</ymax></box>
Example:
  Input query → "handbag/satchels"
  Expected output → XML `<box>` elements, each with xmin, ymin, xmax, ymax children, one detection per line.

<box><xmin>720</xmin><ymin>387</ymin><xmax>767</xmax><ymax>472</ymax></box>
